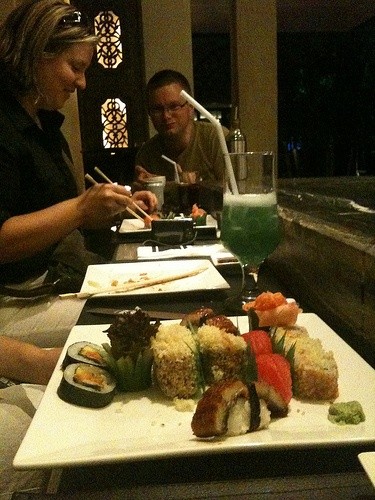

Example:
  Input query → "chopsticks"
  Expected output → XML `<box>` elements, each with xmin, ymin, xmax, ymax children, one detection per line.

<box><xmin>85</xmin><ymin>166</ymin><xmax>153</xmax><ymax>223</ymax></box>
<box><xmin>59</xmin><ymin>267</ymin><xmax>208</xmax><ymax>299</ymax></box>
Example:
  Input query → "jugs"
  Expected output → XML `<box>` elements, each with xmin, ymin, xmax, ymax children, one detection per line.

<box><xmin>228</xmin><ymin>128</ymin><xmax>248</xmax><ymax>180</ymax></box>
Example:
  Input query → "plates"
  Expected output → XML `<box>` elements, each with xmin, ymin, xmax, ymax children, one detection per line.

<box><xmin>12</xmin><ymin>313</ymin><xmax>375</xmax><ymax>488</ymax></box>
<box><xmin>79</xmin><ymin>259</ymin><xmax>230</xmax><ymax>299</ymax></box>
<box><xmin>119</xmin><ymin>214</ymin><xmax>218</xmax><ymax>233</ymax></box>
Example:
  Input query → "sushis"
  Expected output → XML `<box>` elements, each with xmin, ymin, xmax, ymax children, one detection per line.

<box><xmin>57</xmin><ymin>292</ymin><xmax>338</xmax><ymax>438</ymax></box>
<box><xmin>144</xmin><ymin>203</ymin><xmax>206</xmax><ymax>227</ymax></box>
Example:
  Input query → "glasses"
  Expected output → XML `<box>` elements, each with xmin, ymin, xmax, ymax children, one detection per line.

<box><xmin>60</xmin><ymin>11</ymin><xmax>89</xmax><ymax>28</ymax></box>
<box><xmin>148</xmin><ymin>101</ymin><xmax>188</xmax><ymax>118</ymax></box>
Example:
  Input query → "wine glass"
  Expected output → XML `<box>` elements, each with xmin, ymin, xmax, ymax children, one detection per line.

<box><xmin>220</xmin><ymin>151</ymin><xmax>278</xmax><ymax>312</ymax></box>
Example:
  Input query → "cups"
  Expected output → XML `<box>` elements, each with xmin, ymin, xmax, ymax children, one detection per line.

<box><xmin>178</xmin><ymin>171</ymin><xmax>200</xmax><ymax>214</ymax></box>
<box><xmin>142</xmin><ymin>176</ymin><xmax>165</xmax><ymax>206</ymax></box>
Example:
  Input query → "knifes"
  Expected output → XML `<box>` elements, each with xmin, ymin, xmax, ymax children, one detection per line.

<box><xmin>87</xmin><ymin>308</ymin><xmax>187</xmax><ymax>319</ymax></box>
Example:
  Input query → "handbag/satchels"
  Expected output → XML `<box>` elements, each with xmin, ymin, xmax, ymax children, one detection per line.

<box><xmin>0</xmin><ymin>228</ymin><xmax>109</xmax><ymax>297</ymax></box>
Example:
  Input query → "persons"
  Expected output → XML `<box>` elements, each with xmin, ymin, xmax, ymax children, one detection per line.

<box><xmin>0</xmin><ymin>0</ymin><xmax>159</xmax><ymax>290</ymax></box>
<box><xmin>0</xmin><ymin>334</ymin><xmax>64</xmax><ymax>500</ymax></box>
<box><xmin>130</xmin><ymin>69</ymin><xmax>236</xmax><ymax>198</ymax></box>
